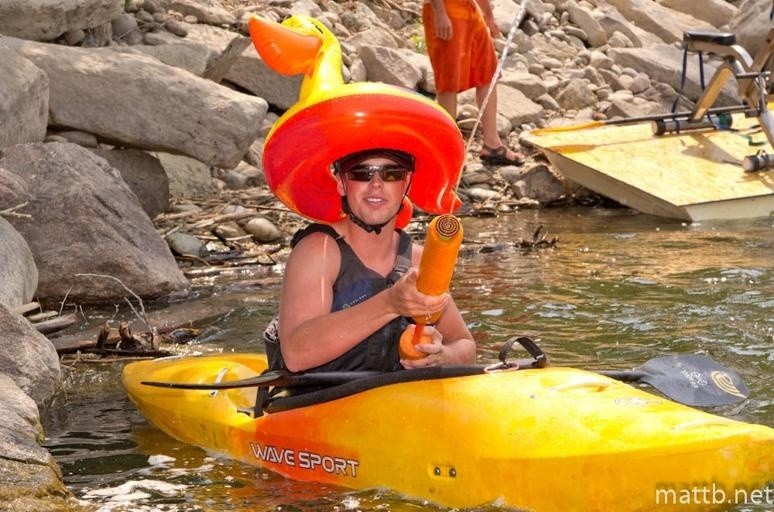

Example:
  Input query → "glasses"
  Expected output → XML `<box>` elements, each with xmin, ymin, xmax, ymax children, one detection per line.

<box><xmin>349</xmin><ymin>165</ymin><xmax>410</xmax><ymax>182</ymax></box>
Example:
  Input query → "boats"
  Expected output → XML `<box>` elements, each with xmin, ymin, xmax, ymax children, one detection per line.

<box><xmin>515</xmin><ymin>22</ymin><xmax>774</xmax><ymax>228</ymax></box>
<box><xmin>115</xmin><ymin>348</ymin><xmax>774</xmax><ymax>512</ymax></box>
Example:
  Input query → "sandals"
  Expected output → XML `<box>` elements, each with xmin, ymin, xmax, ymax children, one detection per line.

<box><xmin>480</xmin><ymin>144</ymin><xmax>525</xmax><ymax>165</ymax></box>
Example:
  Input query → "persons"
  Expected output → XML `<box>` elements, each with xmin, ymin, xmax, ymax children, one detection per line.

<box><xmin>277</xmin><ymin>151</ymin><xmax>476</xmax><ymax>393</ymax></box>
<box><xmin>423</xmin><ymin>0</ymin><xmax>526</xmax><ymax>165</ymax></box>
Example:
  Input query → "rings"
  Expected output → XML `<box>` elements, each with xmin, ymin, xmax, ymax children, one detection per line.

<box><xmin>425</xmin><ymin>312</ymin><xmax>431</xmax><ymax>320</ymax></box>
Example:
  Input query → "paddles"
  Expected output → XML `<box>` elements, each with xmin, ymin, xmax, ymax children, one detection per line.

<box><xmin>530</xmin><ymin>103</ymin><xmax>774</xmax><ymax>135</ymax></box>
<box><xmin>142</xmin><ymin>356</ymin><xmax>746</xmax><ymax>412</ymax></box>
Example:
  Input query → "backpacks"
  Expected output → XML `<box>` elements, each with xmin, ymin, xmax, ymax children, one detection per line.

<box><xmin>264</xmin><ymin>311</ymin><xmax>283</xmax><ymax>373</ymax></box>
